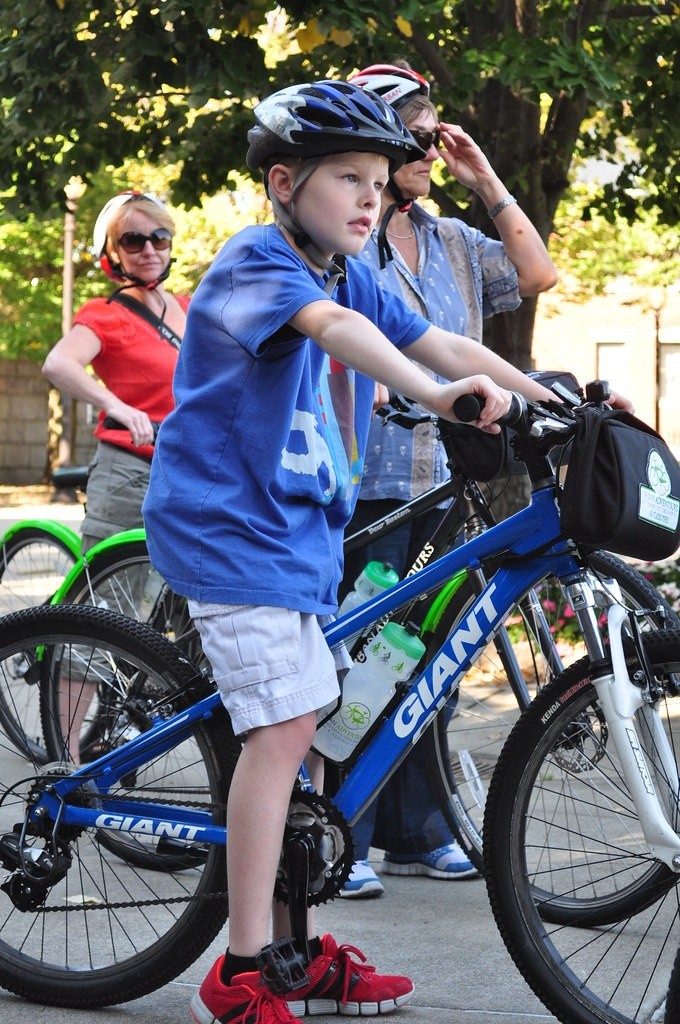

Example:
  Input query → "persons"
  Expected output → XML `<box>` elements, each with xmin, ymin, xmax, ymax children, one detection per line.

<box><xmin>141</xmin><ymin>80</ymin><xmax>636</xmax><ymax>1024</ymax></box>
<box><xmin>324</xmin><ymin>64</ymin><xmax>558</xmax><ymax>898</ymax></box>
<box><xmin>40</xmin><ymin>192</ymin><xmax>190</xmax><ymax>766</ymax></box>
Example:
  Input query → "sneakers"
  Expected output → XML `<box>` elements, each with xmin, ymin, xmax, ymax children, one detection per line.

<box><xmin>382</xmin><ymin>844</ymin><xmax>478</xmax><ymax>878</ymax></box>
<box><xmin>284</xmin><ymin>933</ymin><xmax>415</xmax><ymax>1017</ymax></box>
<box><xmin>334</xmin><ymin>860</ymin><xmax>385</xmax><ymax>897</ymax></box>
<box><xmin>189</xmin><ymin>954</ymin><xmax>305</xmax><ymax>1024</ymax></box>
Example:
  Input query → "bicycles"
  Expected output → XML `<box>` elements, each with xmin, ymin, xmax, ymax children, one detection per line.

<box><xmin>1</xmin><ymin>379</ymin><xmax>680</xmax><ymax>1024</ymax></box>
<box><xmin>0</xmin><ymin>371</ymin><xmax>680</xmax><ymax>926</ymax></box>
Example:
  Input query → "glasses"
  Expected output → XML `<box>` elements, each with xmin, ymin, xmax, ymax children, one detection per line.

<box><xmin>117</xmin><ymin>228</ymin><xmax>173</xmax><ymax>254</ymax></box>
<box><xmin>408</xmin><ymin>130</ymin><xmax>440</xmax><ymax>151</ymax></box>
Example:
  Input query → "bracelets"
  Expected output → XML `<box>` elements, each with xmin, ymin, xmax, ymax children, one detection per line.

<box><xmin>487</xmin><ymin>195</ymin><xmax>517</xmax><ymax>219</ymax></box>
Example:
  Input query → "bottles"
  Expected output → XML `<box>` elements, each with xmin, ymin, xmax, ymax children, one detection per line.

<box><xmin>312</xmin><ymin>620</ymin><xmax>427</xmax><ymax>763</ymax></box>
<box><xmin>338</xmin><ymin>560</ymin><xmax>399</xmax><ymax>664</ymax></box>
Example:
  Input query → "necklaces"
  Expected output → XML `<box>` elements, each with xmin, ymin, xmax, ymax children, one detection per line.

<box><xmin>384</xmin><ymin>223</ymin><xmax>414</xmax><ymax>239</ymax></box>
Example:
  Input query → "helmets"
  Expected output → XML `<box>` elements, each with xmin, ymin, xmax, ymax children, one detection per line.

<box><xmin>93</xmin><ymin>190</ymin><xmax>168</xmax><ymax>258</ymax></box>
<box><xmin>246</xmin><ymin>80</ymin><xmax>427</xmax><ymax>170</ymax></box>
<box><xmin>348</xmin><ymin>63</ymin><xmax>431</xmax><ymax>111</ymax></box>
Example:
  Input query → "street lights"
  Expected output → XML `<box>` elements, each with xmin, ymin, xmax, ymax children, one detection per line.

<box><xmin>50</xmin><ymin>174</ymin><xmax>87</xmax><ymax>504</ymax></box>
